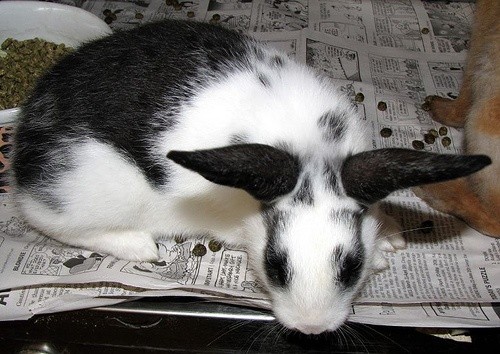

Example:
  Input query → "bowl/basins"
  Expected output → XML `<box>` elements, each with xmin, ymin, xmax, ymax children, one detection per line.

<box><xmin>0</xmin><ymin>0</ymin><xmax>113</xmax><ymax>124</ymax></box>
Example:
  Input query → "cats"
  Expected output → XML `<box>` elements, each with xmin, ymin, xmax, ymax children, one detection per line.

<box><xmin>406</xmin><ymin>1</ymin><xmax>500</xmax><ymax>241</ymax></box>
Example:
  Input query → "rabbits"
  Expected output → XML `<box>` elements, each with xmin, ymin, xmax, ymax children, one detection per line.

<box><xmin>6</xmin><ymin>15</ymin><xmax>491</xmax><ymax>341</ymax></box>
